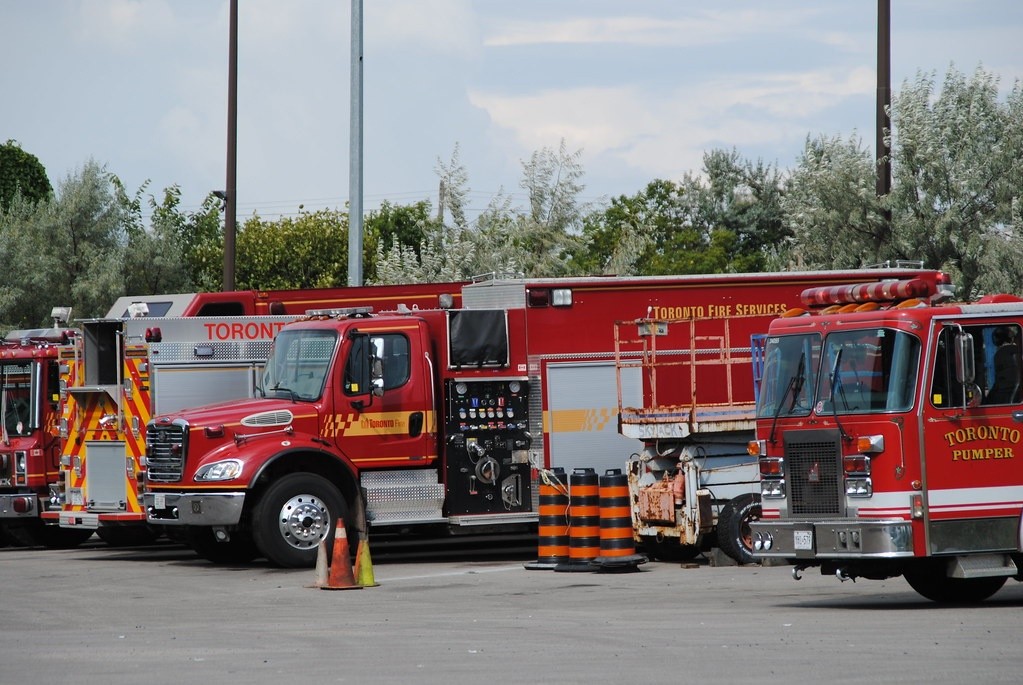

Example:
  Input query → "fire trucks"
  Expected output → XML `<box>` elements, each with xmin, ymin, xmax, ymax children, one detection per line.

<box><xmin>0</xmin><ymin>278</ymin><xmax>479</xmax><ymax>550</ymax></box>
<box><xmin>747</xmin><ymin>278</ymin><xmax>1023</xmax><ymax>607</ymax></box>
<box><xmin>144</xmin><ymin>259</ymin><xmax>952</xmax><ymax>569</ymax></box>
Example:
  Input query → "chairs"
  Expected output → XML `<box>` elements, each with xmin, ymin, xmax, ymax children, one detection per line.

<box><xmin>381</xmin><ymin>352</ymin><xmax>409</xmax><ymax>388</ymax></box>
<box><xmin>985</xmin><ymin>326</ymin><xmax>1021</xmax><ymax>404</ymax></box>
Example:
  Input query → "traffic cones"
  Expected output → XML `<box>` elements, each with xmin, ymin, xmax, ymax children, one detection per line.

<box><xmin>354</xmin><ymin>539</ymin><xmax>380</xmax><ymax>587</ymax></box>
<box><xmin>319</xmin><ymin>518</ymin><xmax>363</xmax><ymax>589</ymax></box>
<box><xmin>304</xmin><ymin>540</ymin><xmax>329</xmax><ymax>588</ymax></box>
